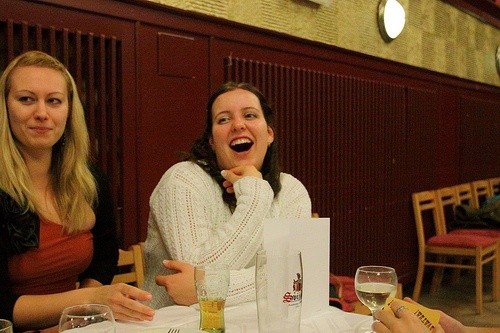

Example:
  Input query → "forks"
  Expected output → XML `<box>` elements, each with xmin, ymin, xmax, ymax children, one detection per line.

<box><xmin>167</xmin><ymin>328</ymin><xmax>180</xmax><ymax>333</ymax></box>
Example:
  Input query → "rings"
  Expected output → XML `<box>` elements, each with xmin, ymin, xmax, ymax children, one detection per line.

<box><xmin>396</xmin><ymin>306</ymin><xmax>406</xmax><ymax>315</ymax></box>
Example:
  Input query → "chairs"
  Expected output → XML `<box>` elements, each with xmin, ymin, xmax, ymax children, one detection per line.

<box><xmin>112</xmin><ymin>245</ymin><xmax>146</xmax><ymax>289</ymax></box>
<box><xmin>411</xmin><ymin>178</ymin><xmax>500</xmax><ymax>315</ymax></box>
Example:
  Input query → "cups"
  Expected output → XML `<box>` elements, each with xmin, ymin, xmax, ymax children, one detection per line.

<box><xmin>58</xmin><ymin>303</ymin><xmax>116</xmax><ymax>333</ymax></box>
<box><xmin>0</xmin><ymin>319</ymin><xmax>13</xmax><ymax>333</ymax></box>
<box><xmin>255</xmin><ymin>249</ymin><xmax>303</xmax><ymax>333</ymax></box>
<box><xmin>193</xmin><ymin>264</ymin><xmax>230</xmax><ymax>333</ymax></box>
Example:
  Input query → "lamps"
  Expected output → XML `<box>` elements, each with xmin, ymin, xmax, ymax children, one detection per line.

<box><xmin>378</xmin><ymin>0</ymin><xmax>406</xmax><ymax>43</ymax></box>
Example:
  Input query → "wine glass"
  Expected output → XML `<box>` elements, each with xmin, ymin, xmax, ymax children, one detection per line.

<box><xmin>354</xmin><ymin>265</ymin><xmax>398</xmax><ymax>333</ymax></box>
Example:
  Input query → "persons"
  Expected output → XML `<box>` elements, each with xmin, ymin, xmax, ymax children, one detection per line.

<box><xmin>0</xmin><ymin>50</ymin><xmax>153</xmax><ymax>333</ymax></box>
<box><xmin>371</xmin><ymin>297</ymin><xmax>500</xmax><ymax>333</ymax></box>
<box><xmin>140</xmin><ymin>82</ymin><xmax>312</xmax><ymax>311</ymax></box>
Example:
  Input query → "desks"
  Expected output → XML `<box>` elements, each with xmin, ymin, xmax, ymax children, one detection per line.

<box><xmin>38</xmin><ymin>298</ymin><xmax>379</xmax><ymax>333</ymax></box>
